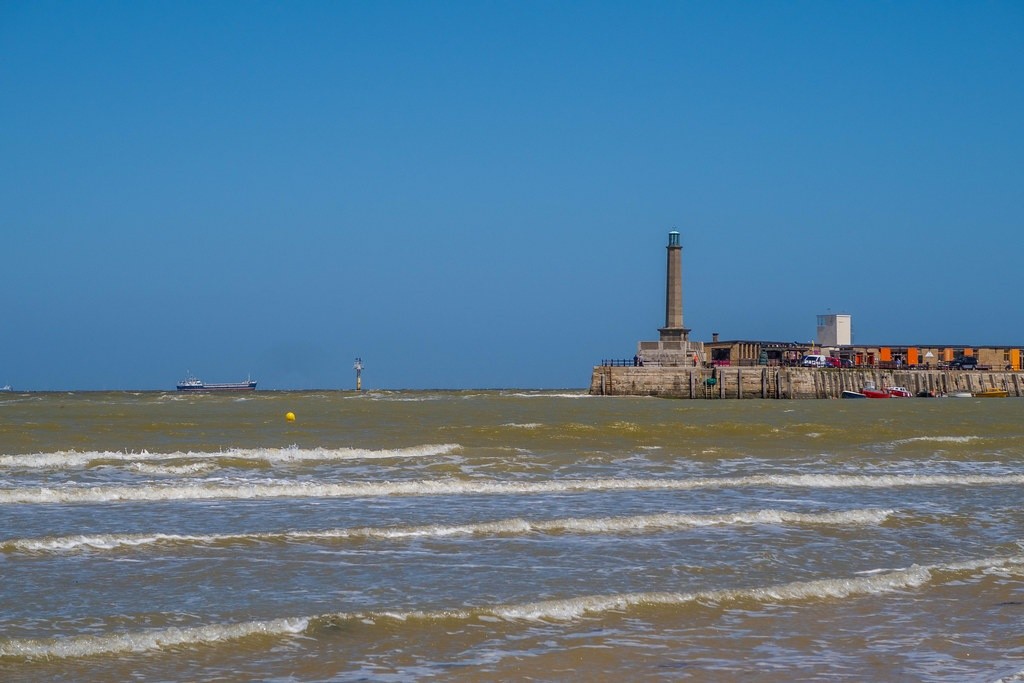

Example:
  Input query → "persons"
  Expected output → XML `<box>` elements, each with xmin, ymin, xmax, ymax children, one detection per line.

<box><xmin>694</xmin><ymin>354</ymin><xmax>698</xmax><ymax>367</ymax></box>
<box><xmin>633</xmin><ymin>354</ymin><xmax>644</xmax><ymax>367</ymax></box>
<box><xmin>896</xmin><ymin>358</ymin><xmax>902</xmax><ymax>369</ymax></box>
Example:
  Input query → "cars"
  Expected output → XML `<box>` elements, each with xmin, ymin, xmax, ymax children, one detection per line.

<box><xmin>936</xmin><ymin>355</ymin><xmax>993</xmax><ymax>372</ymax></box>
<box><xmin>800</xmin><ymin>354</ymin><xmax>855</xmax><ymax>368</ymax></box>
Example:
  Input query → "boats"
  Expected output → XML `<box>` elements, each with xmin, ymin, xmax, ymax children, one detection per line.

<box><xmin>915</xmin><ymin>389</ymin><xmax>935</xmax><ymax>397</ymax></box>
<box><xmin>947</xmin><ymin>389</ymin><xmax>973</xmax><ymax>398</ymax></box>
<box><xmin>885</xmin><ymin>386</ymin><xmax>914</xmax><ymax>398</ymax></box>
<box><xmin>175</xmin><ymin>369</ymin><xmax>258</xmax><ymax>390</ymax></box>
<box><xmin>975</xmin><ymin>387</ymin><xmax>1009</xmax><ymax>398</ymax></box>
<box><xmin>858</xmin><ymin>380</ymin><xmax>892</xmax><ymax>399</ymax></box>
<box><xmin>841</xmin><ymin>390</ymin><xmax>867</xmax><ymax>399</ymax></box>
